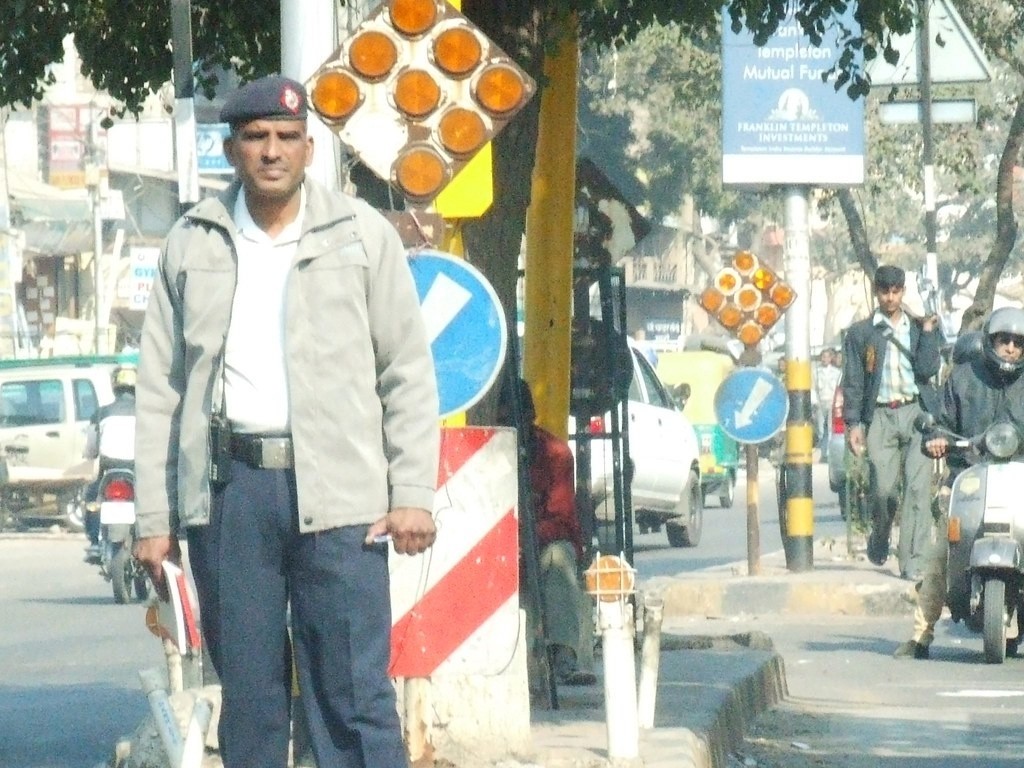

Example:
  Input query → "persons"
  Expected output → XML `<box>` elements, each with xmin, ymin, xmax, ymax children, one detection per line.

<box><xmin>81</xmin><ymin>362</ymin><xmax>138</xmax><ymax>562</ymax></box>
<box><xmin>841</xmin><ymin>265</ymin><xmax>941</xmax><ymax>581</ymax></box>
<box><xmin>893</xmin><ymin>307</ymin><xmax>1024</xmax><ymax>660</ymax></box>
<box><xmin>132</xmin><ymin>76</ymin><xmax>440</xmax><ymax>768</ymax></box>
<box><xmin>496</xmin><ymin>379</ymin><xmax>597</xmax><ymax>685</ymax></box>
<box><xmin>774</xmin><ymin>356</ymin><xmax>786</xmax><ymax>382</ymax></box>
<box><xmin>813</xmin><ymin>349</ymin><xmax>843</xmax><ymax>463</ymax></box>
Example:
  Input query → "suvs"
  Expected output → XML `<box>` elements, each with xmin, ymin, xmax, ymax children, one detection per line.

<box><xmin>0</xmin><ymin>361</ymin><xmax>117</xmax><ymax>531</ymax></box>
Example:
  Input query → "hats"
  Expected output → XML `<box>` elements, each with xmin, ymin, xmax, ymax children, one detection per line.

<box><xmin>218</xmin><ymin>77</ymin><xmax>307</xmax><ymax>121</ymax></box>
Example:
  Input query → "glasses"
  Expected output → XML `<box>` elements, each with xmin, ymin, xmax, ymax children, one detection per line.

<box><xmin>993</xmin><ymin>336</ymin><xmax>1024</xmax><ymax>349</ymax></box>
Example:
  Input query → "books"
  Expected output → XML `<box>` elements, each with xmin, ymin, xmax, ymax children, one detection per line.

<box><xmin>158</xmin><ymin>560</ymin><xmax>200</xmax><ymax>655</ymax></box>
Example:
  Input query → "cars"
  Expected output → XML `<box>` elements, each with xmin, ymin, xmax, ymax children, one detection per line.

<box><xmin>568</xmin><ymin>334</ymin><xmax>747</xmax><ymax>549</ymax></box>
<box><xmin>828</xmin><ymin>376</ymin><xmax>848</xmax><ymax>521</ymax></box>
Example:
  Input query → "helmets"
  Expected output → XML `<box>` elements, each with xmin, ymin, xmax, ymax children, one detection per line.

<box><xmin>110</xmin><ymin>365</ymin><xmax>135</xmax><ymax>396</ymax></box>
<box><xmin>983</xmin><ymin>306</ymin><xmax>1024</xmax><ymax>383</ymax></box>
<box><xmin>952</xmin><ymin>331</ymin><xmax>986</xmax><ymax>362</ymax></box>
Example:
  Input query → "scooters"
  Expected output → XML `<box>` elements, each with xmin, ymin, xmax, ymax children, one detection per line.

<box><xmin>913</xmin><ymin>412</ymin><xmax>1024</xmax><ymax>664</ymax></box>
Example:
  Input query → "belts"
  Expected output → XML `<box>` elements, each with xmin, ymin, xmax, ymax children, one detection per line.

<box><xmin>228</xmin><ymin>433</ymin><xmax>295</xmax><ymax>470</ymax></box>
<box><xmin>876</xmin><ymin>396</ymin><xmax>920</xmax><ymax>408</ymax></box>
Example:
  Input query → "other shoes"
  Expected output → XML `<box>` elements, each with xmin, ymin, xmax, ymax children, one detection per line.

<box><xmin>867</xmin><ymin>532</ymin><xmax>889</xmax><ymax>566</ymax></box>
<box><xmin>893</xmin><ymin>640</ymin><xmax>930</xmax><ymax>659</ymax></box>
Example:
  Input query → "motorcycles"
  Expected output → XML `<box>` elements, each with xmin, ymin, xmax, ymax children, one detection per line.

<box><xmin>84</xmin><ymin>468</ymin><xmax>154</xmax><ymax>603</ymax></box>
